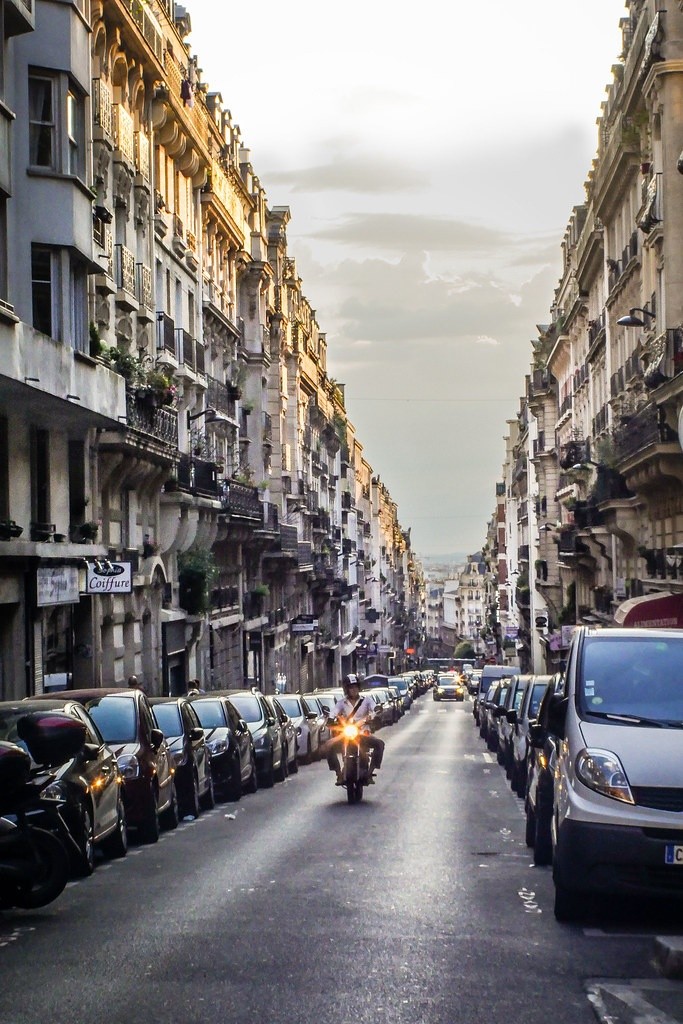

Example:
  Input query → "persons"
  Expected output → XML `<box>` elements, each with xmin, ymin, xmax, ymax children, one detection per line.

<box><xmin>193</xmin><ymin>680</ymin><xmax>206</xmax><ymax>696</ymax></box>
<box><xmin>322</xmin><ymin>674</ymin><xmax>385</xmax><ymax>786</ymax></box>
<box><xmin>183</xmin><ymin>681</ymin><xmax>198</xmax><ymax>697</ymax></box>
<box><xmin>127</xmin><ymin>676</ymin><xmax>147</xmax><ymax>697</ymax></box>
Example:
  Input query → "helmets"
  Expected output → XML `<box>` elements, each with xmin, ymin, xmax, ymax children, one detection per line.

<box><xmin>343</xmin><ymin>673</ymin><xmax>362</xmax><ymax>695</ymax></box>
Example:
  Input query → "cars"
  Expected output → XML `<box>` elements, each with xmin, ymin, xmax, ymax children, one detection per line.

<box><xmin>1</xmin><ymin>607</ymin><xmax>683</xmax><ymax>930</ymax></box>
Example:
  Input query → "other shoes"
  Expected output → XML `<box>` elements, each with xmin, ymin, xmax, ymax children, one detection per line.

<box><xmin>365</xmin><ymin>775</ymin><xmax>377</xmax><ymax>785</ymax></box>
<box><xmin>335</xmin><ymin>774</ymin><xmax>345</xmax><ymax>787</ymax></box>
